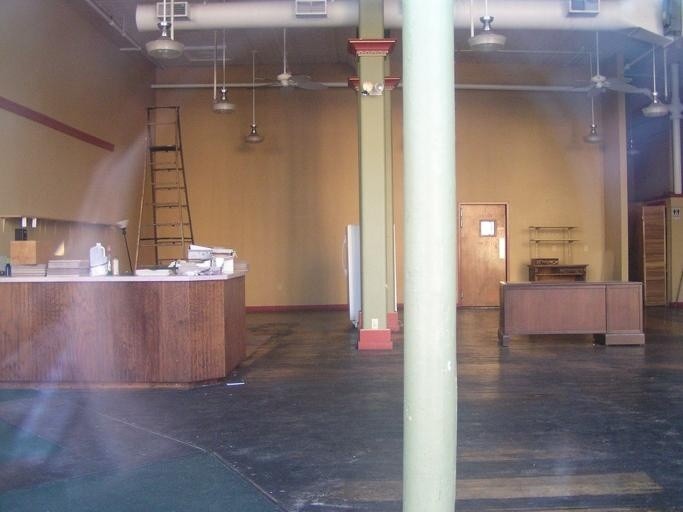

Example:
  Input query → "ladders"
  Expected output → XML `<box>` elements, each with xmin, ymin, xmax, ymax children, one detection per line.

<box><xmin>134</xmin><ymin>105</ymin><xmax>194</xmax><ymax>276</ymax></box>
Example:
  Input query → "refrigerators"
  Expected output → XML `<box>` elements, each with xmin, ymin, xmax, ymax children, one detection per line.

<box><xmin>340</xmin><ymin>224</ymin><xmax>397</xmax><ymax>329</ymax></box>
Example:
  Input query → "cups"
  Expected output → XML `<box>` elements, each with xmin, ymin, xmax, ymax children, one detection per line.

<box><xmin>0</xmin><ymin>271</ymin><xmax>4</xmax><ymax>276</ymax></box>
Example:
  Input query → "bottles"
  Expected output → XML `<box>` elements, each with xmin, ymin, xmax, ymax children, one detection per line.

<box><xmin>105</xmin><ymin>244</ymin><xmax>118</xmax><ymax>276</ymax></box>
<box><xmin>5</xmin><ymin>263</ymin><xmax>10</xmax><ymax>276</ymax></box>
<box><xmin>14</xmin><ymin>229</ymin><xmax>27</xmax><ymax>240</ymax></box>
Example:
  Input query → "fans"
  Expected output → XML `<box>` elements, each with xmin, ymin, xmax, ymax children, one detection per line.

<box><xmin>553</xmin><ymin>28</ymin><xmax>651</xmax><ymax>101</ymax></box>
<box><xmin>252</xmin><ymin>29</ymin><xmax>326</xmax><ymax>91</ymax></box>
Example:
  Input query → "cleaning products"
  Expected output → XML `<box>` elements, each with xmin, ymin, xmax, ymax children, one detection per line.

<box><xmin>107</xmin><ymin>244</ymin><xmax>119</xmax><ymax>276</ymax></box>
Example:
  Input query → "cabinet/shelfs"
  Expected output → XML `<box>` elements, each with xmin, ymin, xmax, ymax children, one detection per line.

<box><xmin>525</xmin><ymin>224</ymin><xmax>582</xmax><ymax>244</ymax></box>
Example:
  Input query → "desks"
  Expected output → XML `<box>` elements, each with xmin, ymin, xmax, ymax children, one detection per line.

<box><xmin>495</xmin><ymin>279</ymin><xmax>644</xmax><ymax>348</ymax></box>
<box><xmin>527</xmin><ymin>264</ymin><xmax>588</xmax><ymax>281</ymax></box>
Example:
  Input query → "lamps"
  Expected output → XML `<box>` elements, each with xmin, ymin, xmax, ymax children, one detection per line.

<box><xmin>468</xmin><ymin>0</ymin><xmax>508</xmax><ymax>55</ymax></box>
<box><xmin>211</xmin><ymin>28</ymin><xmax>238</xmax><ymax>115</ymax></box>
<box><xmin>146</xmin><ymin>0</ymin><xmax>184</xmax><ymax>63</ymax></box>
<box><xmin>641</xmin><ymin>43</ymin><xmax>670</xmax><ymax>119</ymax></box>
<box><xmin>243</xmin><ymin>49</ymin><xmax>263</xmax><ymax>146</ymax></box>
<box><xmin>583</xmin><ymin>97</ymin><xmax>604</xmax><ymax>146</ymax></box>
